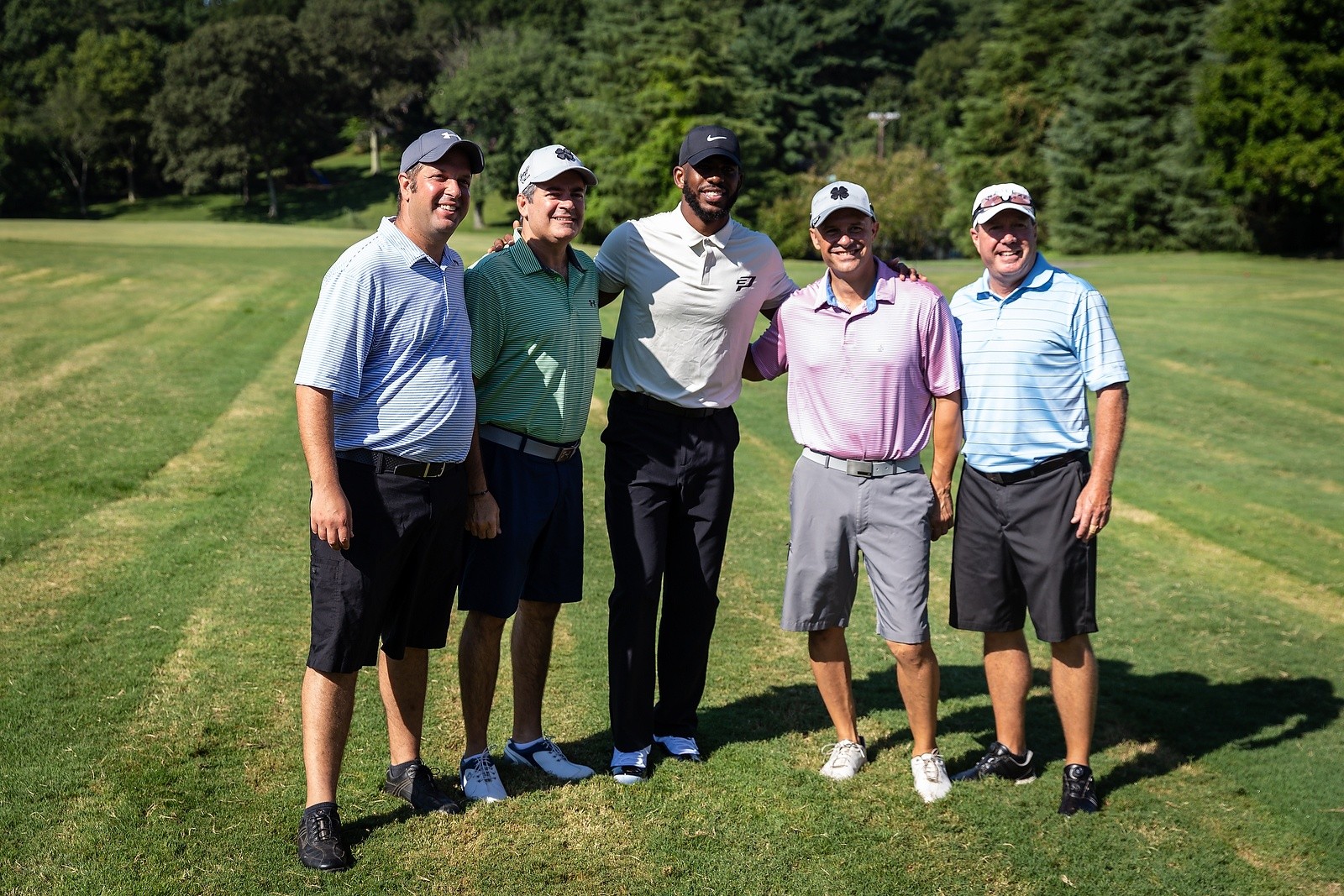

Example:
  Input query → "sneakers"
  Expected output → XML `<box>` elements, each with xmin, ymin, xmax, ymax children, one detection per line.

<box><xmin>950</xmin><ymin>741</ymin><xmax>1037</xmax><ymax>785</ymax></box>
<box><xmin>611</xmin><ymin>741</ymin><xmax>651</xmax><ymax>786</ymax></box>
<box><xmin>297</xmin><ymin>809</ymin><xmax>351</xmax><ymax>870</ymax></box>
<box><xmin>910</xmin><ymin>747</ymin><xmax>951</xmax><ymax>804</ymax></box>
<box><xmin>820</xmin><ymin>736</ymin><xmax>868</xmax><ymax>780</ymax></box>
<box><xmin>503</xmin><ymin>731</ymin><xmax>594</xmax><ymax>780</ymax></box>
<box><xmin>383</xmin><ymin>756</ymin><xmax>460</xmax><ymax>816</ymax></box>
<box><xmin>1059</xmin><ymin>764</ymin><xmax>1102</xmax><ymax>815</ymax></box>
<box><xmin>653</xmin><ymin>731</ymin><xmax>702</xmax><ymax>762</ymax></box>
<box><xmin>460</xmin><ymin>745</ymin><xmax>507</xmax><ymax>803</ymax></box>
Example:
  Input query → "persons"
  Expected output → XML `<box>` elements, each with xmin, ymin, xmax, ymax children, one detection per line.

<box><xmin>294</xmin><ymin>127</ymin><xmax>487</xmax><ymax>865</ymax></box>
<box><xmin>742</xmin><ymin>181</ymin><xmax>960</xmax><ymax>804</ymax></box>
<box><xmin>947</xmin><ymin>183</ymin><xmax>1131</xmax><ymax>814</ymax></box>
<box><xmin>490</xmin><ymin>127</ymin><xmax>928</xmax><ymax>782</ymax></box>
<box><xmin>455</xmin><ymin>143</ymin><xmax>615</xmax><ymax>805</ymax></box>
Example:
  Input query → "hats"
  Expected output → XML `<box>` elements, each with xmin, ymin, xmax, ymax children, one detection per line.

<box><xmin>517</xmin><ymin>144</ymin><xmax>598</xmax><ymax>194</ymax></box>
<box><xmin>972</xmin><ymin>183</ymin><xmax>1037</xmax><ymax>228</ymax></box>
<box><xmin>679</xmin><ymin>126</ymin><xmax>740</xmax><ymax>167</ymax></box>
<box><xmin>809</xmin><ymin>181</ymin><xmax>876</xmax><ymax>227</ymax></box>
<box><xmin>399</xmin><ymin>129</ymin><xmax>484</xmax><ymax>174</ymax></box>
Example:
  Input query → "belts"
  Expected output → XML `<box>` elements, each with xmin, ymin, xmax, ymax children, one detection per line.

<box><xmin>969</xmin><ymin>449</ymin><xmax>1086</xmax><ymax>486</ymax></box>
<box><xmin>803</xmin><ymin>445</ymin><xmax>920</xmax><ymax>478</ymax></box>
<box><xmin>615</xmin><ymin>388</ymin><xmax>721</xmax><ymax>419</ymax></box>
<box><xmin>335</xmin><ymin>445</ymin><xmax>464</xmax><ymax>478</ymax></box>
<box><xmin>478</xmin><ymin>422</ymin><xmax>581</xmax><ymax>462</ymax></box>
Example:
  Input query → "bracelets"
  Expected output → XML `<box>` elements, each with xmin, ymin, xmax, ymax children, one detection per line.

<box><xmin>475</xmin><ymin>491</ymin><xmax>489</xmax><ymax>497</ymax></box>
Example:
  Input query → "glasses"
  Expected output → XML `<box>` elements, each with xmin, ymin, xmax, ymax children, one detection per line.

<box><xmin>971</xmin><ymin>191</ymin><xmax>1036</xmax><ymax>227</ymax></box>
